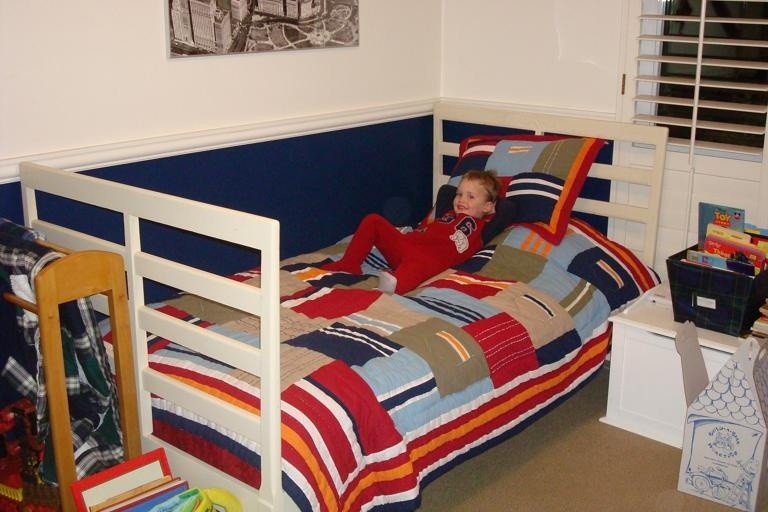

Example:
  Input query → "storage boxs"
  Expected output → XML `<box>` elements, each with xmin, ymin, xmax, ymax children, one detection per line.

<box><xmin>674</xmin><ymin>319</ymin><xmax>767</xmax><ymax>512</ymax></box>
<box><xmin>666</xmin><ymin>232</ymin><xmax>768</xmax><ymax>337</ymax></box>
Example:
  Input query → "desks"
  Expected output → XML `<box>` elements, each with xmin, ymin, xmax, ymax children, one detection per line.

<box><xmin>599</xmin><ymin>285</ymin><xmax>746</xmax><ymax>451</ymax></box>
<box><xmin>19</xmin><ymin>102</ymin><xmax>669</xmax><ymax>512</ymax></box>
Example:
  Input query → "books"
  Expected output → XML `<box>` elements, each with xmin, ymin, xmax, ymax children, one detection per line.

<box><xmin>682</xmin><ymin>204</ymin><xmax>768</xmax><ymax>277</ymax></box>
<box><xmin>95</xmin><ymin>476</ymin><xmax>190</xmax><ymax>512</ymax></box>
<box><xmin>751</xmin><ymin>303</ymin><xmax>768</xmax><ymax>338</ymax></box>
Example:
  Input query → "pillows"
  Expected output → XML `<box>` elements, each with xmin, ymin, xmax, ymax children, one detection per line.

<box><xmin>423</xmin><ymin>135</ymin><xmax>605</xmax><ymax>244</ymax></box>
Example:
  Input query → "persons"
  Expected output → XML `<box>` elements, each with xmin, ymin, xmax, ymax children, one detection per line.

<box><xmin>319</xmin><ymin>167</ymin><xmax>519</xmax><ymax>298</ymax></box>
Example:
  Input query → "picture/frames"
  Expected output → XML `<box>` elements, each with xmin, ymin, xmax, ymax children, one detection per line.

<box><xmin>68</xmin><ymin>447</ymin><xmax>172</xmax><ymax>512</ymax></box>
